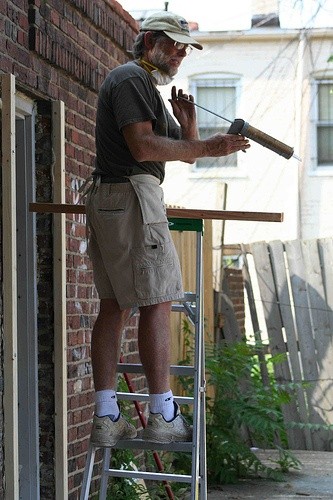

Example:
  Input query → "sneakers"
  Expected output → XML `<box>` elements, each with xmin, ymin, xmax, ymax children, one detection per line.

<box><xmin>145</xmin><ymin>400</ymin><xmax>192</xmax><ymax>444</ymax></box>
<box><xmin>90</xmin><ymin>402</ymin><xmax>137</xmax><ymax>447</ymax></box>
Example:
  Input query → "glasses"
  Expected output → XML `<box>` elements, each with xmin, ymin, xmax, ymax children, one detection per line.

<box><xmin>153</xmin><ymin>31</ymin><xmax>192</xmax><ymax>56</ymax></box>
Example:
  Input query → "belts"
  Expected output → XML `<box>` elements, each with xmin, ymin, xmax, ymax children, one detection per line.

<box><xmin>93</xmin><ymin>175</ymin><xmax>131</xmax><ymax>183</ymax></box>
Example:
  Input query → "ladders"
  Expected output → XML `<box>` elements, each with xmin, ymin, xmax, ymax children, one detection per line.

<box><xmin>76</xmin><ymin>216</ymin><xmax>210</xmax><ymax>500</ymax></box>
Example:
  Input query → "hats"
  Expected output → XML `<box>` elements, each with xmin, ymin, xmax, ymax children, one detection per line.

<box><xmin>139</xmin><ymin>11</ymin><xmax>203</xmax><ymax>51</ymax></box>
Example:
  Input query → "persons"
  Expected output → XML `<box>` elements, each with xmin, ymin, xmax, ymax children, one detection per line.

<box><xmin>83</xmin><ymin>7</ymin><xmax>251</xmax><ymax>449</ymax></box>
